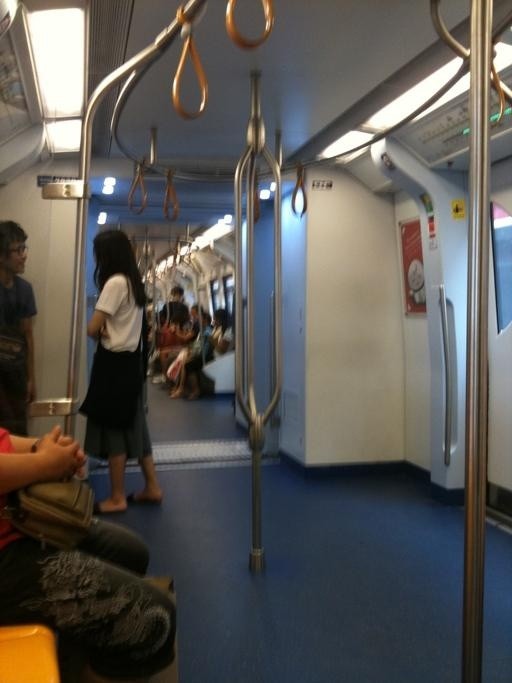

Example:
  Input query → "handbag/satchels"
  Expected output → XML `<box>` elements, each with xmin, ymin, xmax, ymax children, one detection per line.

<box><xmin>7</xmin><ymin>471</ymin><xmax>97</xmax><ymax>552</ymax></box>
<box><xmin>76</xmin><ymin>342</ymin><xmax>144</xmax><ymax>431</ymax></box>
<box><xmin>0</xmin><ymin>332</ymin><xmax>30</xmax><ymax>372</ymax></box>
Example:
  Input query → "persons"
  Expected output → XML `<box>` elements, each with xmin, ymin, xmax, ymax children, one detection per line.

<box><xmin>143</xmin><ymin>283</ymin><xmax>234</xmax><ymax>402</ymax></box>
<box><xmin>1</xmin><ymin>219</ymin><xmax>38</xmax><ymax>436</ymax></box>
<box><xmin>77</xmin><ymin>228</ymin><xmax>164</xmax><ymax>516</ymax></box>
<box><xmin>0</xmin><ymin>421</ymin><xmax>182</xmax><ymax>683</ymax></box>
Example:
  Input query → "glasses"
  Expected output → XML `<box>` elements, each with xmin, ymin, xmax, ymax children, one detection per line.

<box><xmin>7</xmin><ymin>245</ymin><xmax>29</xmax><ymax>255</ymax></box>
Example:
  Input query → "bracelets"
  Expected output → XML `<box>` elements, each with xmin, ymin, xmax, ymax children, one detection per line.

<box><xmin>30</xmin><ymin>438</ymin><xmax>44</xmax><ymax>454</ymax></box>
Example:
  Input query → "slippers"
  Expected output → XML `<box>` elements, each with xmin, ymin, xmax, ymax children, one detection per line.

<box><xmin>123</xmin><ymin>492</ymin><xmax>163</xmax><ymax>507</ymax></box>
